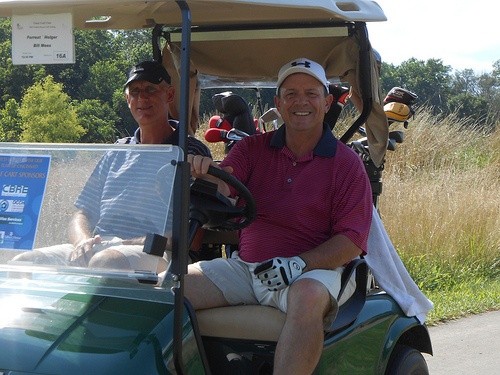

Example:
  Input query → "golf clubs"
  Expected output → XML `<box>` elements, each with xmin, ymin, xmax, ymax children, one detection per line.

<box><xmin>205</xmin><ymin>91</ymin><xmax>280</xmax><ymax>154</ymax></box>
<box><xmin>350</xmin><ymin>87</ymin><xmax>418</xmax><ymax>161</ymax></box>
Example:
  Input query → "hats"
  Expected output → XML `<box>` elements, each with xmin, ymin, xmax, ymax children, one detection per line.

<box><xmin>275</xmin><ymin>58</ymin><xmax>329</xmax><ymax>93</ymax></box>
<box><xmin>123</xmin><ymin>61</ymin><xmax>171</xmax><ymax>90</ymax></box>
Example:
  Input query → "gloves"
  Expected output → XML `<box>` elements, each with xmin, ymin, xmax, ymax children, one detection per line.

<box><xmin>253</xmin><ymin>254</ymin><xmax>306</xmax><ymax>291</ymax></box>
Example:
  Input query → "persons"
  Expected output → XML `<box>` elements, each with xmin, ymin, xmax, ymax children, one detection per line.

<box><xmin>12</xmin><ymin>61</ymin><xmax>212</xmax><ymax>275</ymax></box>
<box><xmin>157</xmin><ymin>59</ymin><xmax>373</xmax><ymax>375</ymax></box>
